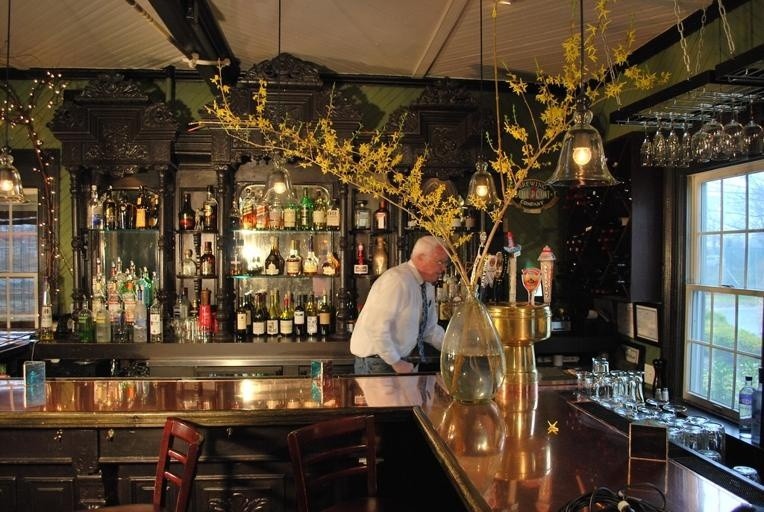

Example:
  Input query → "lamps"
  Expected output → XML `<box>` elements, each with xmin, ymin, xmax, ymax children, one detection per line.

<box><xmin>0</xmin><ymin>1</ymin><xmax>25</xmax><ymax>201</ymax></box>
<box><xmin>465</xmin><ymin>1</ymin><xmax>506</xmax><ymax>210</ymax></box>
<box><xmin>545</xmin><ymin>0</ymin><xmax>626</xmax><ymax>189</ymax></box>
<box><xmin>261</xmin><ymin>3</ymin><xmax>296</xmax><ymax>193</ymax></box>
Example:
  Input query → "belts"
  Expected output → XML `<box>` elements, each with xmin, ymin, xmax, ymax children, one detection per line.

<box><xmin>366</xmin><ymin>353</ymin><xmax>381</xmax><ymax>359</ymax></box>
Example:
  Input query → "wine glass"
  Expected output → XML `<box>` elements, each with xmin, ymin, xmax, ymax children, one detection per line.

<box><xmin>641</xmin><ymin>93</ymin><xmax>763</xmax><ymax>168</ymax></box>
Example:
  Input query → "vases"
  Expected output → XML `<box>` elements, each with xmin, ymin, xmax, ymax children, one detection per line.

<box><xmin>439</xmin><ymin>297</ymin><xmax>506</xmax><ymax>405</ymax></box>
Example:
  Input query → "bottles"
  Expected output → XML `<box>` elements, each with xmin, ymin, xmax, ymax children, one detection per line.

<box><xmin>38</xmin><ymin>275</ymin><xmax>59</xmax><ymax>340</ymax></box>
<box><xmin>738</xmin><ymin>376</ymin><xmax>754</xmax><ymax>432</ymax></box>
<box><xmin>437</xmin><ymin>266</ymin><xmax>457</xmax><ymax>323</ymax></box>
<box><xmin>752</xmin><ymin>366</ymin><xmax>764</xmax><ymax>444</ymax></box>
<box><xmin>76</xmin><ymin>184</ymin><xmax>386</xmax><ymax>343</ymax></box>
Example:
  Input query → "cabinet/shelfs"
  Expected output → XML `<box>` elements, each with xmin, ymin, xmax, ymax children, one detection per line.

<box><xmin>163</xmin><ymin>160</ymin><xmax>227</xmax><ymax>335</ymax></box>
<box><xmin>350</xmin><ymin>168</ymin><xmax>404</xmax><ymax>337</ymax></box>
<box><xmin>71</xmin><ymin>158</ymin><xmax>163</xmax><ymax>331</ymax></box>
<box><xmin>228</xmin><ymin>158</ymin><xmax>351</xmax><ymax>336</ymax></box>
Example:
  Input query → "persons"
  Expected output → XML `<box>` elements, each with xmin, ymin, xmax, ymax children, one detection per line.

<box><xmin>350</xmin><ymin>236</ymin><xmax>448</xmax><ymax>374</ymax></box>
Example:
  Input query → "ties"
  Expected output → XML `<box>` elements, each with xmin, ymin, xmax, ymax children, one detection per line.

<box><xmin>417</xmin><ymin>280</ymin><xmax>428</xmax><ymax>364</ymax></box>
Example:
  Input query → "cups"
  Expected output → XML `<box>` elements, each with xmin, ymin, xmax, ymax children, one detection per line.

<box><xmin>577</xmin><ymin>357</ymin><xmax>728</xmax><ymax>458</ymax></box>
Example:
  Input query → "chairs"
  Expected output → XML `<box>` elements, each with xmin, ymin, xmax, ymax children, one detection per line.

<box><xmin>285</xmin><ymin>414</ymin><xmax>382</xmax><ymax>509</ymax></box>
<box><xmin>93</xmin><ymin>414</ymin><xmax>208</xmax><ymax>511</ymax></box>
